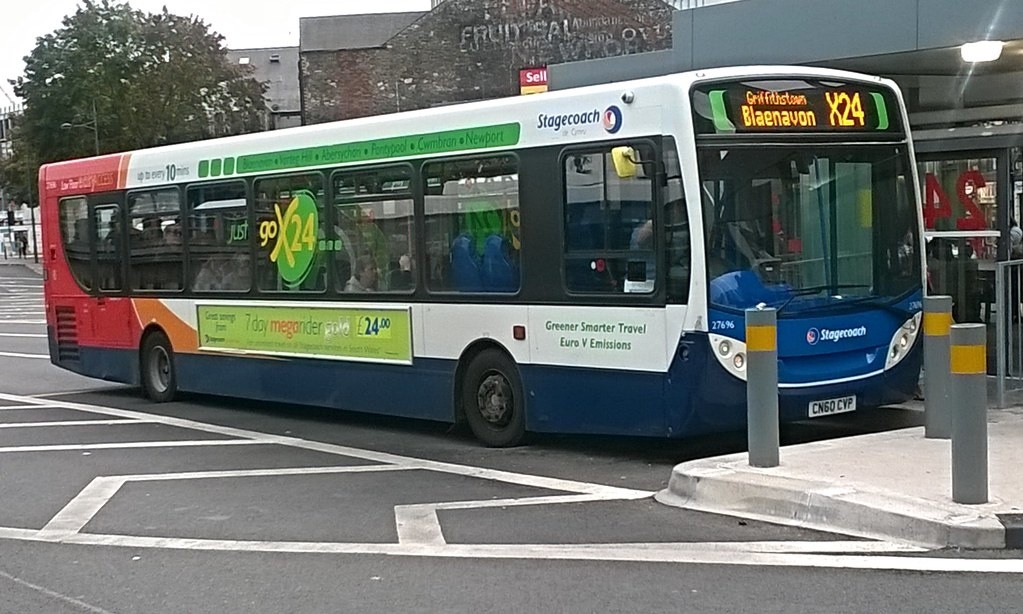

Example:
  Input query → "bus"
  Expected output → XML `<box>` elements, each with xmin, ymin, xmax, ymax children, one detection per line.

<box><xmin>37</xmin><ymin>66</ymin><xmax>927</xmax><ymax>448</ymax></box>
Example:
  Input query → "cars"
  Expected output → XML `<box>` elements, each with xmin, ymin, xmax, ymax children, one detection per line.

<box><xmin>171</xmin><ymin>199</ymin><xmax>246</xmax><ymax>236</ymax></box>
<box><xmin>134</xmin><ymin>220</ymin><xmax>175</xmax><ymax>235</ymax></box>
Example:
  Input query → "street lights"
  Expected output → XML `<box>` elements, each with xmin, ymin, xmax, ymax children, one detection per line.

<box><xmin>61</xmin><ymin>123</ymin><xmax>99</xmax><ymax>155</ymax></box>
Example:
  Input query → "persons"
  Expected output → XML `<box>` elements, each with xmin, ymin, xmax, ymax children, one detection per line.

<box><xmin>927</xmin><ymin>217</ymin><xmax>1023</xmax><ymax>324</ymax></box>
<box><xmin>344</xmin><ymin>255</ymin><xmax>378</xmax><ymax>292</ymax></box>
<box><xmin>629</xmin><ymin>220</ymin><xmax>655</xmax><ymax>249</ymax></box>
<box><xmin>20</xmin><ymin>236</ymin><xmax>28</xmax><ymax>260</ymax></box>
<box><xmin>390</xmin><ymin>254</ymin><xmax>417</xmax><ymax>292</ymax></box>
<box><xmin>195</xmin><ymin>254</ymin><xmax>251</xmax><ymax>288</ymax></box>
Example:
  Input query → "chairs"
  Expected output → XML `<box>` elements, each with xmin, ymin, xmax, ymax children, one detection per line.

<box><xmin>320</xmin><ymin>249</ymin><xmax>413</xmax><ymax>293</ymax></box>
<box><xmin>447</xmin><ymin>232</ymin><xmax>519</xmax><ymax>292</ymax></box>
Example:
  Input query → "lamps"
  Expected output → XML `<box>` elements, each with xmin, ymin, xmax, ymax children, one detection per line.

<box><xmin>961</xmin><ymin>40</ymin><xmax>1003</xmax><ymax>61</ymax></box>
